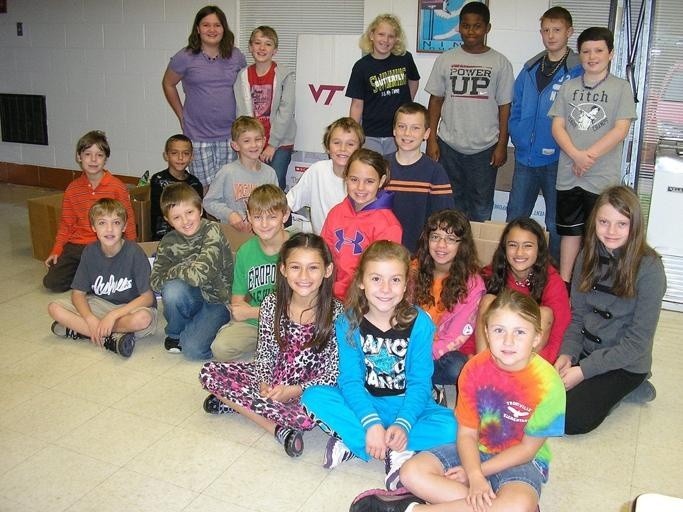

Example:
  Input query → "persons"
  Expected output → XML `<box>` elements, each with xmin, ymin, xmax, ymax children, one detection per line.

<box><xmin>320</xmin><ymin>147</ymin><xmax>404</xmax><ymax>302</ymax></box>
<box><xmin>163</xmin><ymin>6</ymin><xmax>248</xmax><ymax>221</ymax></box>
<box><xmin>345</xmin><ymin>14</ymin><xmax>420</xmax><ymax>156</ymax></box>
<box><xmin>380</xmin><ymin>102</ymin><xmax>454</xmax><ymax>255</ymax></box>
<box><xmin>403</xmin><ymin>208</ymin><xmax>486</xmax><ymax>407</ymax></box>
<box><xmin>350</xmin><ymin>289</ymin><xmax>565</xmax><ymax>510</ymax></box>
<box><xmin>48</xmin><ymin>198</ymin><xmax>159</xmax><ymax>357</ymax></box>
<box><xmin>148</xmin><ymin>183</ymin><xmax>235</xmax><ymax>359</ymax></box>
<box><xmin>202</xmin><ymin>115</ymin><xmax>279</xmax><ymax>251</ymax></box>
<box><xmin>507</xmin><ymin>6</ymin><xmax>584</xmax><ymax>261</ymax></box>
<box><xmin>234</xmin><ymin>26</ymin><xmax>297</xmax><ymax>193</ymax></box>
<box><xmin>285</xmin><ymin>117</ymin><xmax>366</xmax><ymax>235</ymax></box>
<box><xmin>44</xmin><ymin>130</ymin><xmax>136</xmax><ymax>294</ymax></box>
<box><xmin>422</xmin><ymin>2</ymin><xmax>514</xmax><ymax>222</ymax></box>
<box><xmin>211</xmin><ymin>183</ymin><xmax>294</xmax><ymax>362</ymax></box>
<box><xmin>200</xmin><ymin>233</ymin><xmax>343</xmax><ymax>456</ymax></box>
<box><xmin>150</xmin><ymin>135</ymin><xmax>204</xmax><ymax>240</ymax></box>
<box><xmin>553</xmin><ymin>185</ymin><xmax>666</xmax><ymax>434</ymax></box>
<box><xmin>547</xmin><ymin>27</ymin><xmax>638</xmax><ymax>297</ymax></box>
<box><xmin>300</xmin><ymin>238</ymin><xmax>458</xmax><ymax>492</ymax></box>
<box><xmin>460</xmin><ymin>218</ymin><xmax>571</xmax><ymax>363</ymax></box>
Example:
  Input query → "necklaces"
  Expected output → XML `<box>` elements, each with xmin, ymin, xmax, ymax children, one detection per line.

<box><xmin>582</xmin><ymin>71</ymin><xmax>609</xmax><ymax>89</ymax></box>
<box><xmin>541</xmin><ymin>46</ymin><xmax>570</xmax><ymax>77</ymax></box>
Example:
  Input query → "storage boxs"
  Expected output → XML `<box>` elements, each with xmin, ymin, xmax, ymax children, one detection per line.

<box><xmin>465</xmin><ymin>220</ymin><xmax>548</xmax><ymax>267</ymax></box>
<box><xmin>127</xmin><ymin>188</ymin><xmax>152</xmax><ymax>242</ymax></box>
<box><xmin>27</xmin><ymin>194</ymin><xmax>63</xmax><ymax>263</ymax></box>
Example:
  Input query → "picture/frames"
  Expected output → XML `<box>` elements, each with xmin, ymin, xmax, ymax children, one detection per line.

<box><xmin>415</xmin><ymin>0</ymin><xmax>490</xmax><ymax>53</ymax></box>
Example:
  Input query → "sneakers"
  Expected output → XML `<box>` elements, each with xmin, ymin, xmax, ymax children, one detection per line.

<box><xmin>275</xmin><ymin>424</ymin><xmax>305</xmax><ymax>458</ymax></box>
<box><xmin>103</xmin><ymin>330</ymin><xmax>138</xmax><ymax>358</ymax></box>
<box><xmin>50</xmin><ymin>319</ymin><xmax>86</xmax><ymax>340</ymax></box>
<box><xmin>383</xmin><ymin>445</ymin><xmax>417</xmax><ymax>493</ymax></box>
<box><xmin>321</xmin><ymin>435</ymin><xmax>355</xmax><ymax>470</ymax></box>
<box><xmin>202</xmin><ymin>394</ymin><xmax>236</xmax><ymax>414</ymax></box>
<box><xmin>348</xmin><ymin>488</ymin><xmax>426</xmax><ymax>512</ymax></box>
<box><xmin>621</xmin><ymin>379</ymin><xmax>658</xmax><ymax>405</ymax></box>
<box><xmin>162</xmin><ymin>335</ymin><xmax>184</xmax><ymax>355</ymax></box>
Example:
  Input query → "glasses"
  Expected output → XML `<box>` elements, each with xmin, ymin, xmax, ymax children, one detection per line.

<box><xmin>428</xmin><ymin>232</ymin><xmax>462</xmax><ymax>245</ymax></box>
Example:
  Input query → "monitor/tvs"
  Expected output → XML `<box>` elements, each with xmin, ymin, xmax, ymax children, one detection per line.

<box><xmin>0</xmin><ymin>92</ymin><xmax>49</xmax><ymax>146</ymax></box>
